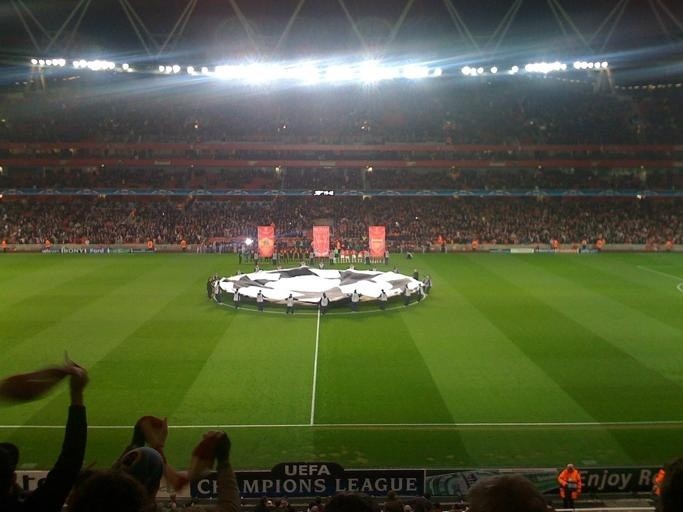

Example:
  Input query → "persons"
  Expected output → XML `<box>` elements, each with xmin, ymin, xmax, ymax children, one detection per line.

<box><xmin>393</xmin><ymin>264</ymin><xmax>399</xmax><ymax>273</ymax></box>
<box><xmin>556</xmin><ymin>463</ymin><xmax>583</xmax><ymax>508</ymax></box>
<box><xmin>308</xmin><ymin>490</ymin><xmax>466</xmax><ymax>511</ymax></box>
<box><xmin>348</xmin><ymin>264</ymin><xmax>360</xmax><ymax>270</ymax></box>
<box><xmin>371</xmin><ymin>265</ymin><xmax>377</xmax><ymax>272</ymax></box>
<box><xmin>259</xmin><ymin>495</ymin><xmax>289</xmax><ymax>508</ymax></box>
<box><xmin>401</xmin><ymin>283</ymin><xmax>412</xmax><ymax>307</ymax></box>
<box><xmin>413</xmin><ymin>268</ymin><xmax>419</xmax><ymax>280</ymax></box>
<box><xmin>467</xmin><ymin>474</ymin><xmax>549</xmax><ymax>511</ymax></box>
<box><xmin>234</xmin><ymin>269</ymin><xmax>247</xmax><ymax>275</ymax></box>
<box><xmin>318</xmin><ymin>262</ymin><xmax>325</xmax><ymax>269</ymax></box>
<box><xmin>236</xmin><ymin>249</ymin><xmax>390</xmax><ymax>264</ymax></box>
<box><xmin>70</xmin><ymin>361</ymin><xmax>242</xmax><ymax>511</ymax></box>
<box><xmin>0</xmin><ymin>86</ymin><xmax>683</xmax><ymax>252</ymax></box>
<box><xmin>1</xmin><ymin>350</ymin><xmax>96</xmax><ymax>505</ymax></box>
<box><xmin>253</xmin><ymin>265</ymin><xmax>264</xmax><ymax>272</ymax></box>
<box><xmin>320</xmin><ymin>291</ymin><xmax>333</xmax><ymax>316</ymax></box>
<box><xmin>347</xmin><ymin>289</ymin><xmax>362</xmax><ymax>312</ymax></box>
<box><xmin>377</xmin><ymin>288</ymin><xmax>388</xmax><ymax>312</ymax></box>
<box><xmin>231</xmin><ymin>288</ymin><xmax>249</xmax><ymax>310</ymax></box>
<box><xmin>425</xmin><ymin>273</ymin><xmax>433</xmax><ymax>295</ymax></box>
<box><xmin>256</xmin><ymin>290</ymin><xmax>267</xmax><ymax>312</ymax></box>
<box><xmin>416</xmin><ymin>283</ymin><xmax>424</xmax><ymax>304</ymax></box>
<box><xmin>284</xmin><ymin>294</ymin><xmax>299</xmax><ymax>315</ymax></box>
<box><xmin>650</xmin><ymin>458</ymin><xmax>682</xmax><ymax>510</ymax></box>
<box><xmin>205</xmin><ymin>272</ymin><xmax>227</xmax><ymax>305</ymax></box>
